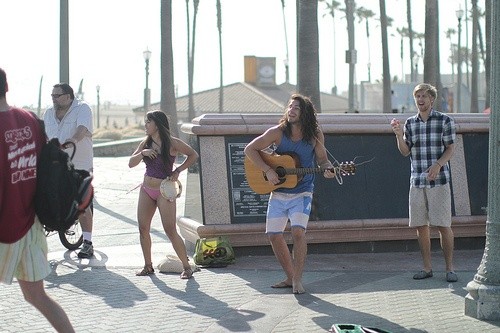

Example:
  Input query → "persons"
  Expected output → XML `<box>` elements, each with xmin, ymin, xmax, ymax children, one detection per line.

<box><xmin>43</xmin><ymin>83</ymin><xmax>94</xmax><ymax>257</ymax></box>
<box><xmin>0</xmin><ymin>67</ymin><xmax>74</xmax><ymax>333</ymax></box>
<box><xmin>244</xmin><ymin>94</ymin><xmax>335</xmax><ymax>293</ymax></box>
<box><xmin>128</xmin><ymin>110</ymin><xmax>199</xmax><ymax>279</ymax></box>
<box><xmin>390</xmin><ymin>84</ymin><xmax>458</xmax><ymax>282</ymax></box>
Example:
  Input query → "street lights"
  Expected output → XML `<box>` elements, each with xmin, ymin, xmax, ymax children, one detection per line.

<box><xmin>96</xmin><ymin>86</ymin><xmax>99</xmax><ymax>128</ymax></box>
<box><xmin>457</xmin><ymin>10</ymin><xmax>464</xmax><ymax>113</ymax></box>
<box><xmin>142</xmin><ymin>51</ymin><xmax>151</xmax><ymax>112</ymax></box>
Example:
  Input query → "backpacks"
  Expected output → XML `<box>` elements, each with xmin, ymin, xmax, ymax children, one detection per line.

<box><xmin>27</xmin><ymin>112</ymin><xmax>95</xmax><ymax>232</ymax></box>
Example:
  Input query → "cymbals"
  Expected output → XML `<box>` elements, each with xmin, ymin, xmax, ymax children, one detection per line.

<box><xmin>159</xmin><ymin>176</ymin><xmax>184</xmax><ymax>202</ymax></box>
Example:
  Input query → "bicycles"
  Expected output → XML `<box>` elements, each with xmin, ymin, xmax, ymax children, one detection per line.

<box><xmin>43</xmin><ymin>202</ymin><xmax>94</xmax><ymax>250</ymax></box>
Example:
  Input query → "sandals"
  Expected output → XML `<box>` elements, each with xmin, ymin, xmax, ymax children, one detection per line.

<box><xmin>180</xmin><ymin>266</ymin><xmax>193</xmax><ymax>279</ymax></box>
<box><xmin>136</xmin><ymin>262</ymin><xmax>155</xmax><ymax>276</ymax></box>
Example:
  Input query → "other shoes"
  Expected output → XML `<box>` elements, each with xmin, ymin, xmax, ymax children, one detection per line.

<box><xmin>413</xmin><ymin>269</ymin><xmax>433</xmax><ymax>279</ymax></box>
<box><xmin>446</xmin><ymin>272</ymin><xmax>458</xmax><ymax>282</ymax></box>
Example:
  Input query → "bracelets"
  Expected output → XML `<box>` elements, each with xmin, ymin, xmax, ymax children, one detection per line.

<box><xmin>175</xmin><ymin>168</ymin><xmax>180</xmax><ymax>174</ymax></box>
<box><xmin>264</xmin><ymin>168</ymin><xmax>273</xmax><ymax>174</ymax></box>
<box><xmin>141</xmin><ymin>151</ymin><xmax>144</xmax><ymax>157</ymax></box>
<box><xmin>435</xmin><ymin>161</ymin><xmax>442</xmax><ymax>167</ymax></box>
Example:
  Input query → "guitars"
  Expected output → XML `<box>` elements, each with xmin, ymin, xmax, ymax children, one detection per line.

<box><xmin>244</xmin><ymin>151</ymin><xmax>358</xmax><ymax>195</ymax></box>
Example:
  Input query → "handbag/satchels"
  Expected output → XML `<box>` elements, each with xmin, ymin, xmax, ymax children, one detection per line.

<box><xmin>157</xmin><ymin>254</ymin><xmax>198</xmax><ymax>274</ymax></box>
<box><xmin>193</xmin><ymin>236</ymin><xmax>236</xmax><ymax>265</ymax></box>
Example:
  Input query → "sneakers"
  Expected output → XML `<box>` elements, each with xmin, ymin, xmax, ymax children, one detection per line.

<box><xmin>78</xmin><ymin>239</ymin><xmax>94</xmax><ymax>258</ymax></box>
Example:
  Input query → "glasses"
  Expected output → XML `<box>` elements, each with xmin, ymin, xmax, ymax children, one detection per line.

<box><xmin>51</xmin><ymin>93</ymin><xmax>68</xmax><ymax>98</ymax></box>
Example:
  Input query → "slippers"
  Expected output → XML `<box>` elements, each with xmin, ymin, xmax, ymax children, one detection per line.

<box><xmin>292</xmin><ymin>285</ymin><xmax>306</xmax><ymax>294</ymax></box>
<box><xmin>271</xmin><ymin>281</ymin><xmax>293</xmax><ymax>288</ymax></box>
